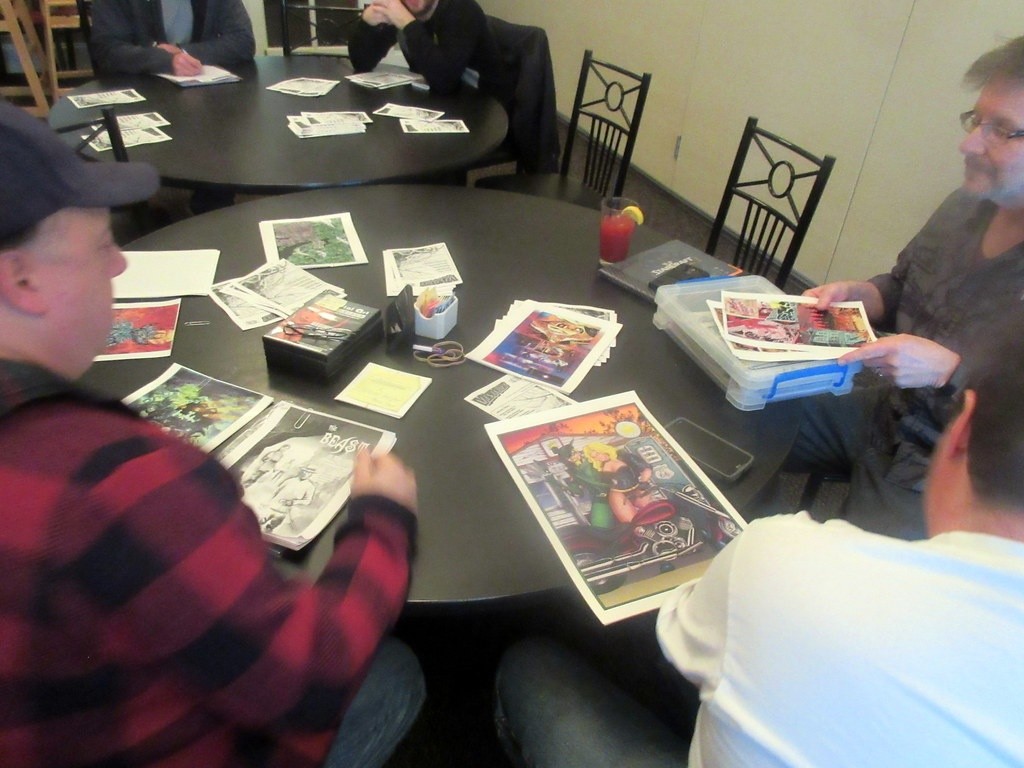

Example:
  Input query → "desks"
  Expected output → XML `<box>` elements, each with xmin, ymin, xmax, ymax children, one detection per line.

<box><xmin>47</xmin><ymin>54</ymin><xmax>510</xmax><ymax>225</ymax></box>
<box><xmin>80</xmin><ymin>185</ymin><xmax>803</xmax><ymax>622</ymax></box>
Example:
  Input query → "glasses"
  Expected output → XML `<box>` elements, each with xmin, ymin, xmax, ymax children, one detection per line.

<box><xmin>960</xmin><ymin>110</ymin><xmax>1024</xmax><ymax>146</ymax></box>
<box><xmin>283</xmin><ymin>323</ymin><xmax>347</xmax><ymax>342</ymax></box>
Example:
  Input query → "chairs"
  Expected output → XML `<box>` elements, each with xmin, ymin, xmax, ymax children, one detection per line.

<box><xmin>51</xmin><ymin>107</ymin><xmax>172</xmax><ymax>247</ymax></box>
<box><xmin>279</xmin><ymin>0</ymin><xmax>371</xmax><ymax>59</ymax></box>
<box><xmin>441</xmin><ymin>16</ymin><xmax>560</xmax><ymax>186</ymax></box>
<box><xmin>472</xmin><ymin>49</ymin><xmax>652</xmax><ymax>210</ymax></box>
<box><xmin>704</xmin><ymin>115</ymin><xmax>837</xmax><ymax>290</ymax></box>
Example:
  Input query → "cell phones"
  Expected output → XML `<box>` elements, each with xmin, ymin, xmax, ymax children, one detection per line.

<box><xmin>665</xmin><ymin>417</ymin><xmax>755</xmax><ymax>483</ymax></box>
<box><xmin>648</xmin><ymin>262</ymin><xmax>710</xmax><ymax>291</ymax></box>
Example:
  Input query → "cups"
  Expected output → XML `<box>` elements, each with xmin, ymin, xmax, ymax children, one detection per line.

<box><xmin>598</xmin><ymin>197</ymin><xmax>641</xmax><ymax>267</ymax></box>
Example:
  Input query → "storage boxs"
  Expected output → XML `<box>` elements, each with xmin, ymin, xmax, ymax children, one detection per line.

<box><xmin>653</xmin><ymin>274</ymin><xmax>862</xmax><ymax>412</ymax></box>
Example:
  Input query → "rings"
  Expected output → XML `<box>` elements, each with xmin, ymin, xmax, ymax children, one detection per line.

<box><xmin>876</xmin><ymin>368</ymin><xmax>882</xmax><ymax>378</ymax></box>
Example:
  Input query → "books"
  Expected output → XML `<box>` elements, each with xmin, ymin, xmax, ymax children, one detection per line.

<box><xmin>597</xmin><ymin>241</ymin><xmax>750</xmax><ymax>307</ymax></box>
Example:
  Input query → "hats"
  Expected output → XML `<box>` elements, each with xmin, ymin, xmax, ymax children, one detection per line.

<box><xmin>0</xmin><ymin>101</ymin><xmax>160</xmax><ymax>241</ymax></box>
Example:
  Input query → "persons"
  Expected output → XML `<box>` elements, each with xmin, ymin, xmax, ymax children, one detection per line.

<box><xmin>89</xmin><ymin>0</ymin><xmax>255</xmax><ymax>229</ymax></box>
<box><xmin>348</xmin><ymin>0</ymin><xmax>499</xmax><ymax>187</ymax></box>
<box><xmin>556</xmin><ymin>442</ymin><xmax>653</xmax><ymax>529</ymax></box>
<box><xmin>780</xmin><ymin>33</ymin><xmax>1024</xmax><ymax>542</ymax></box>
<box><xmin>486</xmin><ymin>310</ymin><xmax>1024</xmax><ymax>768</ymax></box>
<box><xmin>0</xmin><ymin>97</ymin><xmax>425</xmax><ymax>768</ymax></box>
